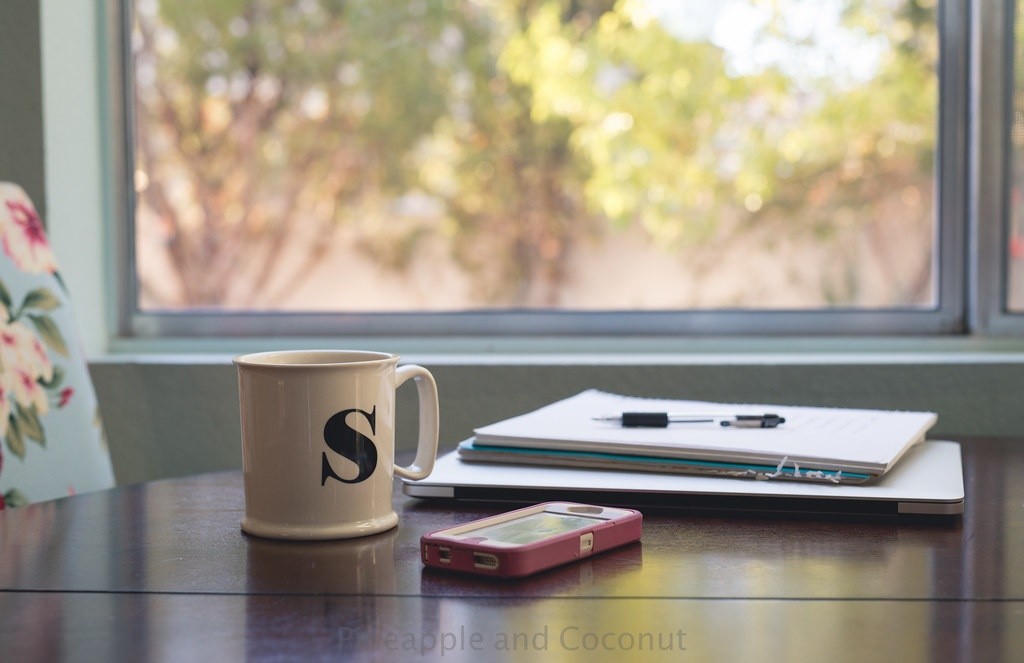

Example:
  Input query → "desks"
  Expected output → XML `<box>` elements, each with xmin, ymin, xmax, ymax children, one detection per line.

<box><xmin>0</xmin><ymin>439</ymin><xmax>992</xmax><ymax>663</ymax></box>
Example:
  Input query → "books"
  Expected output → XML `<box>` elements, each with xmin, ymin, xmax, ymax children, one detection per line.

<box><xmin>458</xmin><ymin>389</ymin><xmax>937</xmax><ymax>485</ymax></box>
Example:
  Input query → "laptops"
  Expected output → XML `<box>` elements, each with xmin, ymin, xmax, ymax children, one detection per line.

<box><xmin>401</xmin><ymin>441</ymin><xmax>967</xmax><ymax>517</ymax></box>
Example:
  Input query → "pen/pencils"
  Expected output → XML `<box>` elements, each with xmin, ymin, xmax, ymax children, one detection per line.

<box><xmin>589</xmin><ymin>411</ymin><xmax>787</xmax><ymax>429</ymax></box>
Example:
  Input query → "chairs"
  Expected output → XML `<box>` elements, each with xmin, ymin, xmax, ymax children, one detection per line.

<box><xmin>0</xmin><ymin>181</ymin><xmax>118</xmax><ymax>509</ymax></box>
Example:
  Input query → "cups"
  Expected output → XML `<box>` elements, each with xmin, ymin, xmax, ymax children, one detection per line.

<box><xmin>233</xmin><ymin>350</ymin><xmax>439</xmax><ymax>540</ymax></box>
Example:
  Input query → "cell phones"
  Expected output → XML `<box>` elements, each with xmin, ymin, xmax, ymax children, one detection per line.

<box><xmin>422</xmin><ymin>500</ymin><xmax>644</xmax><ymax>579</ymax></box>
